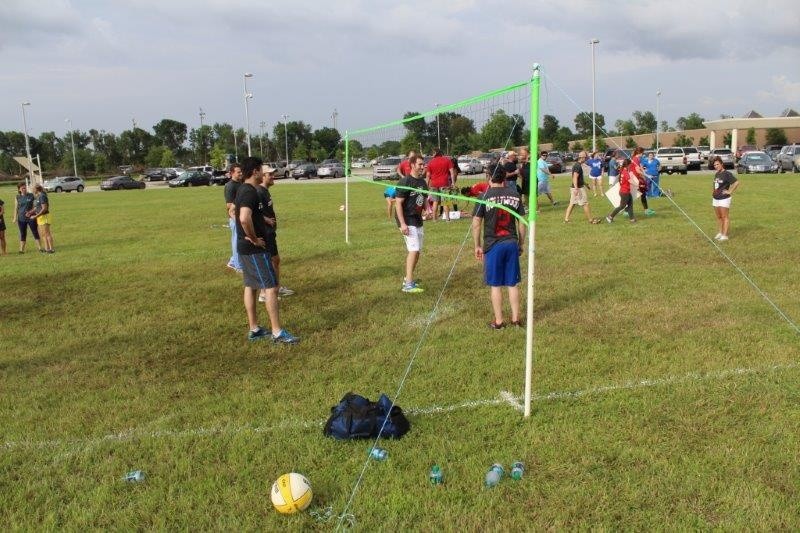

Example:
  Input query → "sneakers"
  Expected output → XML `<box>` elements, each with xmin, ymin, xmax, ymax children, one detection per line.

<box><xmin>278</xmin><ymin>286</ymin><xmax>295</xmax><ymax>296</ymax></box>
<box><xmin>258</xmin><ymin>293</ymin><xmax>282</xmax><ymax>304</ymax></box>
<box><xmin>271</xmin><ymin>328</ymin><xmax>301</xmax><ymax>344</ymax></box>
<box><xmin>248</xmin><ymin>326</ymin><xmax>272</xmax><ymax>340</ymax></box>
<box><xmin>401</xmin><ymin>277</ymin><xmax>425</xmax><ymax>292</ymax></box>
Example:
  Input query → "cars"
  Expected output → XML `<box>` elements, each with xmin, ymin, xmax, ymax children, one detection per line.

<box><xmin>707</xmin><ymin>149</ymin><xmax>735</xmax><ymax>171</ymax></box>
<box><xmin>169</xmin><ymin>171</ymin><xmax>214</xmax><ymax>188</ymax></box>
<box><xmin>351</xmin><ymin>160</ymin><xmax>372</xmax><ymax>168</ymax></box>
<box><xmin>765</xmin><ymin>145</ymin><xmax>790</xmax><ymax>160</ymax></box>
<box><xmin>683</xmin><ymin>147</ymin><xmax>702</xmax><ymax>171</ymax></box>
<box><xmin>43</xmin><ymin>176</ymin><xmax>86</xmax><ymax>193</ymax></box>
<box><xmin>735</xmin><ymin>146</ymin><xmax>758</xmax><ymax>161</ymax></box>
<box><xmin>122</xmin><ymin>163</ymin><xmax>240</xmax><ymax>186</ymax></box>
<box><xmin>736</xmin><ymin>150</ymin><xmax>779</xmax><ymax>173</ymax></box>
<box><xmin>477</xmin><ymin>152</ymin><xmax>498</xmax><ymax>170</ymax></box>
<box><xmin>456</xmin><ymin>154</ymin><xmax>485</xmax><ymax>175</ymax></box>
<box><xmin>545</xmin><ymin>150</ymin><xmax>656</xmax><ymax>175</ymax></box>
<box><xmin>262</xmin><ymin>159</ymin><xmax>352</xmax><ymax>180</ymax></box>
<box><xmin>776</xmin><ymin>145</ymin><xmax>800</xmax><ymax>173</ymax></box>
<box><xmin>100</xmin><ymin>176</ymin><xmax>146</xmax><ymax>191</ymax></box>
<box><xmin>698</xmin><ymin>145</ymin><xmax>711</xmax><ymax>159</ymax></box>
<box><xmin>655</xmin><ymin>147</ymin><xmax>688</xmax><ymax>175</ymax></box>
<box><xmin>372</xmin><ymin>157</ymin><xmax>404</xmax><ymax>181</ymax></box>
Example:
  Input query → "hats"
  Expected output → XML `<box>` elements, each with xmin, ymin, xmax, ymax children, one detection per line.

<box><xmin>508</xmin><ymin>151</ymin><xmax>518</xmax><ymax>155</ymax></box>
<box><xmin>579</xmin><ymin>151</ymin><xmax>590</xmax><ymax>159</ymax></box>
<box><xmin>262</xmin><ymin>164</ymin><xmax>277</xmax><ymax>174</ymax></box>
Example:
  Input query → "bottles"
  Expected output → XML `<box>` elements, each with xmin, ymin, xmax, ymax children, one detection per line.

<box><xmin>485</xmin><ymin>462</ymin><xmax>504</xmax><ymax>488</ymax></box>
<box><xmin>124</xmin><ymin>470</ymin><xmax>146</xmax><ymax>483</ymax></box>
<box><xmin>511</xmin><ymin>461</ymin><xmax>524</xmax><ymax>480</ymax></box>
<box><xmin>367</xmin><ymin>447</ymin><xmax>389</xmax><ymax>462</ymax></box>
<box><xmin>430</xmin><ymin>465</ymin><xmax>442</xmax><ymax>487</ymax></box>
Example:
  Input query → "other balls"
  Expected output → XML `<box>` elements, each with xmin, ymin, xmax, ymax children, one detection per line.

<box><xmin>339</xmin><ymin>204</ymin><xmax>345</xmax><ymax>211</ymax></box>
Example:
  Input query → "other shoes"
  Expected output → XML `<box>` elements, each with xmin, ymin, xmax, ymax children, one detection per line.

<box><xmin>619</xmin><ymin>209</ymin><xmax>630</xmax><ymax>217</ymax></box>
<box><xmin>16</xmin><ymin>251</ymin><xmax>24</xmax><ymax>256</ymax></box>
<box><xmin>714</xmin><ymin>233</ymin><xmax>729</xmax><ymax>241</ymax></box>
<box><xmin>39</xmin><ymin>249</ymin><xmax>43</xmax><ymax>254</ymax></box>
<box><xmin>553</xmin><ymin>201</ymin><xmax>560</xmax><ymax>207</ymax></box>
<box><xmin>601</xmin><ymin>192</ymin><xmax>604</xmax><ymax>196</ymax></box>
<box><xmin>45</xmin><ymin>249</ymin><xmax>55</xmax><ymax>253</ymax></box>
<box><xmin>605</xmin><ymin>215</ymin><xmax>614</xmax><ymax>223</ymax></box>
<box><xmin>487</xmin><ymin>320</ymin><xmax>506</xmax><ymax>330</ymax></box>
<box><xmin>592</xmin><ymin>218</ymin><xmax>601</xmax><ymax>224</ymax></box>
<box><xmin>645</xmin><ymin>209</ymin><xmax>656</xmax><ymax>216</ymax></box>
<box><xmin>506</xmin><ymin>319</ymin><xmax>521</xmax><ymax>327</ymax></box>
<box><xmin>227</xmin><ymin>260</ymin><xmax>243</xmax><ymax>273</ymax></box>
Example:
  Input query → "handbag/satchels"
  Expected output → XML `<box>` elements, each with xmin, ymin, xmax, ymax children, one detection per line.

<box><xmin>638</xmin><ymin>178</ymin><xmax>649</xmax><ymax>193</ymax></box>
<box><xmin>323</xmin><ymin>392</ymin><xmax>410</xmax><ymax>441</ymax></box>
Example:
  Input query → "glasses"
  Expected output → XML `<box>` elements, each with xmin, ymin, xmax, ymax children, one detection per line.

<box><xmin>542</xmin><ymin>155</ymin><xmax>547</xmax><ymax>156</ymax></box>
<box><xmin>269</xmin><ymin>172</ymin><xmax>274</xmax><ymax>176</ymax></box>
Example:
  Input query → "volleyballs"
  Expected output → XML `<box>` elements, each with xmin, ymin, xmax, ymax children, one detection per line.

<box><xmin>271</xmin><ymin>472</ymin><xmax>314</xmax><ymax>515</ymax></box>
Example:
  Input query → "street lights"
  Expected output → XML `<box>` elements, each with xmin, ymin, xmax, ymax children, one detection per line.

<box><xmin>64</xmin><ymin>118</ymin><xmax>79</xmax><ymax>177</ymax></box>
<box><xmin>589</xmin><ymin>38</ymin><xmax>601</xmax><ymax>154</ymax></box>
<box><xmin>21</xmin><ymin>102</ymin><xmax>36</xmax><ymax>189</ymax></box>
<box><xmin>243</xmin><ymin>72</ymin><xmax>253</xmax><ymax>159</ymax></box>
<box><xmin>258</xmin><ymin>121</ymin><xmax>268</xmax><ymax>157</ymax></box>
<box><xmin>281</xmin><ymin>114</ymin><xmax>292</xmax><ymax>165</ymax></box>
<box><xmin>656</xmin><ymin>91</ymin><xmax>664</xmax><ymax>150</ymax></box>
<box><xmin>434</xmin><ymin>103</ymin><xmax>440</xmax><ymax>150</ymax></box>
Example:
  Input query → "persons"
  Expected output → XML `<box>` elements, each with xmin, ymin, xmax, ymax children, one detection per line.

<box><xmin>224</xmin><ymin>157</ymin><xmax>302</xmax><ymax>345</ymax></box>
<box><xmin>0</xmin><ymin>182</ymin><xmax>56</xmax><ymax>255</ymax></box>
<box><xmin>382</xmin><ymin>146</ymin><xmax>743</xmax><ymax>331</ymax></box>
